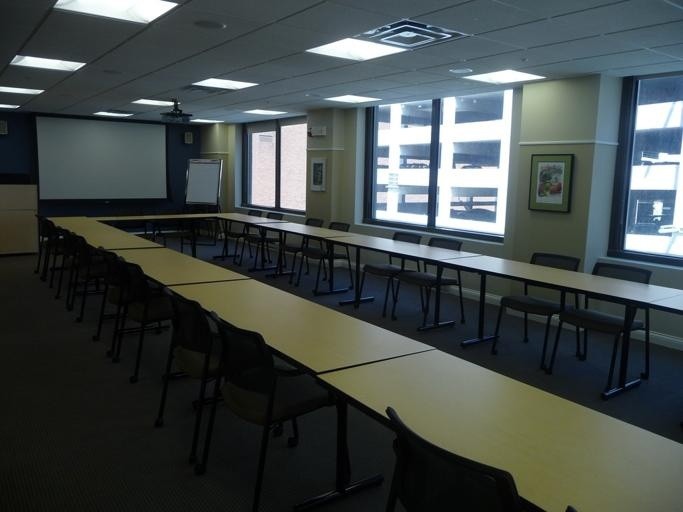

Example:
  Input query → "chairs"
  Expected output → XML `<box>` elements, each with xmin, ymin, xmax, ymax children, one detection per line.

<box><xmin>281</xmin><ymin>218</ymin><xmax>327</xmax><ymax>286</ymax></box>
<box><xmin>238</xmin><ymin>213</ymin><xmax>287</xmax><ymax>267</ymax></box>
<box><xmin>543</xmin><ymin>262</ymin><xmax>653</xmax><ymax>379</ymax></box>
<box><xmin>391</xmin><ymin>238</ymin><xmax>465</xmax><ymax>325</ymax></box>
<box><xmin>55</xmin><ymin>230</ymin><xmax>99</xmax><ymax>308</ymax></box>
<box><xmin>354</xmin><ymin>232</ymin><xmax>425</xmax><ymax>316</ymax></box>
<box><xmin>42</xmin><ymin>220</ymin><xmax>55</xmax><ymax>288</ymax></box>
<box><xmin>73</xmin><ymin>237</ymin><xmax>107</xmax><ymax>322</ymax></box>
<box><xmin>294</xmin><ymin>222</ymin><xmax>354</xmax><ymax>290</ymax></box>
<box><xmin>115</xmin><ymin>257</ymin><xmax>172</xmax><ymax>383</ymax></box>
<box><xmin>386</xmin><ymin>405</ymin><xmax>542</xmax><ymax>512</ymax></box>
<box><xmin>491</xmin><ymin>253</ymin><xmax>583</xmax><ymax>368</ymax></box>
<box><xmin>155</xmin><ymin>289</ymin><xmax>285</xmax><ymax>464</ymax></box>
<box><xmin>34</xmin><ymin>214</ymin><xmax>47</xmax><ymax>273</ymax></box>
<box><xmin>223</xmin><ymin>210</ymin><xmax>271</xmax><ymax>264</ymax></box>
<box><xmin>92</xmin><ymin>251</ymin><xmax>162</xmax><ymax>355</ymax></box>
<box><xmin>194</xmin><ymin>310</ymin><xmax>351</xmax><ymax>511</ymax></box>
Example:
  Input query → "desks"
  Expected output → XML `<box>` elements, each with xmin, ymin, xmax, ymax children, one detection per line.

<box><xmin>418</xmin><ymin>255</ymin><xmax>683</xmax><ymax>400</ymax></box>
<box><xmin>89</xmin><ymin>215</ymin><xmax>166</xmax><ymax>246</ymax></box>
<box><xmin>326</xmin><ymin>237</ymin><xmax>481</xmax><ymax>330</ymax></box>
<box><xmin>106</xmin><ymin>248</ymin><xmax>251</xmax><ymax>387</ymax></box>
<box><xmin>168</xmin><ymin>280</ymin><xmax>436</xmax><ymax>512</ymax></box>
<box><xmin>650</xmin><ymin>291</ymin><xmax>683</xmax><ymax>316</ymax></box>
<box><xmin>145</xmin><ymin>213</ymin><xmax>240</xmax><ymax>259</ymax></box>
<box><xmin>48</xmin><ymin>216</ymin><xmax>166</xmax><ymax>319</ymax></box>
<box><xmin>256</xmin><ymin>222</ymin><xmax>373</xmax><ymax>296</ymax></box>
<box><xmin>317</xmin><ymin>350</ymin><xmax>682</xmax><ymax>512</ymax></box>
<box><xmin>212</xmin><ymin>214</ymin><xmax>290</xmax><ymax>271</ymax></box>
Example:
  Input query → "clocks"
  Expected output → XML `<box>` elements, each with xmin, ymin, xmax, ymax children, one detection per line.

<box><xmin>0</xmin><ymin>120</ymin><xmax>8</xmax><ymax>135</ymax></box>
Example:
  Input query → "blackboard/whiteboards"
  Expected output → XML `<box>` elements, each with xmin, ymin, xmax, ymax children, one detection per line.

<box><xmin>185</xmin><ymin>158</ymin><xmax>223</xmax><ymax>206</ymax></box>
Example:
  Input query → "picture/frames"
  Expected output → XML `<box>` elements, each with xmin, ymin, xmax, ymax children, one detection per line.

<box><xmin>528</xmin><ymin>153</ymin><xmax>576</xmax><ymax>213</ymax></box>
<box><xmin>310</xmin><ymin>157</ymin><xmax>327</xmax><ymax>191</ymax></box>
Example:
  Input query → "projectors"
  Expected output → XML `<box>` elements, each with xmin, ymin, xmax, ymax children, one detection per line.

<box><xmin>161</xmin><ymin>115</ymin><xmax>190</xmax><ymax>123</ymax></box>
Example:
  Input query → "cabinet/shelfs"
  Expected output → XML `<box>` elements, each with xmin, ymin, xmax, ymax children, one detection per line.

<box><xmin>1</xmin><ymin>184</ymin><xmax>40</xmax><ymax>255</ymax></box>
